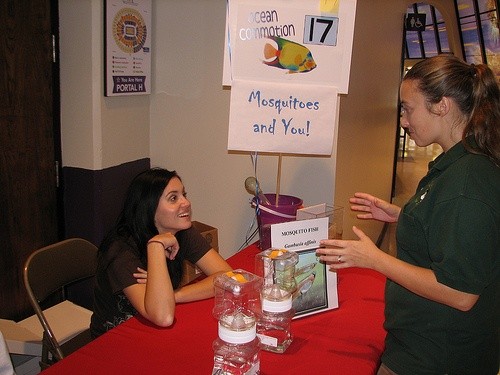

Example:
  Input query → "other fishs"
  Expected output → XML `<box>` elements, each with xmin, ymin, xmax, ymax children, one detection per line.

<box><xmin>292</xmin><ymin>272</ymin><xmax>316</xmax><ymax>302</ymax></box>
<box><xmin>295</xmin><ymin>262</ymin><xmax>317</xmax><ymax>276</ymax></box>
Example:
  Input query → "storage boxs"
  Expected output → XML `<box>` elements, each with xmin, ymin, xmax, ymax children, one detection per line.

<box><xmin>179</xmin><ymin>221</ymin><xmax>219</xmax><ymax>287</ymax></box>
<box><xmin>264</xmin><ymin>217</ymin><xmax>339</xmax><ymax>320</ymax></box>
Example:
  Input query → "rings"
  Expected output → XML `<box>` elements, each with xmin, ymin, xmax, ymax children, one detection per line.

<box><xmin>337</xmin><ymin>255</ymin><xmax>342</xmax><ymax>263</ymax></box>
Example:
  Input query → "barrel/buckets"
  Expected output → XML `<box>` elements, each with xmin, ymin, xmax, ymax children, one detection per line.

<box><xmin>249</xmin><ymin>194</ymin><xmax>303</xmax><ymax>251</ymax></box>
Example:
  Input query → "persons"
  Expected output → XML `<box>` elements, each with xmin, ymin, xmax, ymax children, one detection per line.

<box><xmin>316</xmin><ymin>54</ymin><xmax>500</xmax><ymax>375</ymax></box>
<box><xmin>90</xmin><ymin>167</ymin><xmax>236</xmax><ymax>339</ymax></box>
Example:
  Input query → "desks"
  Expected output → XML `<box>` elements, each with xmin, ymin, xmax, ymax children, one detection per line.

<box><xmin>38</xmin><ymin>239</ymin><xmax>389</xmax><ymax>375</ymax></box>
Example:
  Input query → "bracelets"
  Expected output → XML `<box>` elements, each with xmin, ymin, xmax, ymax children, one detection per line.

<box><xmin>147</xmin><ymin>240</ymin><xmax>165</xmax><ymax>250</ymax></box>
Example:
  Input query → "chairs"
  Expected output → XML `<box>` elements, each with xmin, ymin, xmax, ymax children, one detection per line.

<box><xmin>23</xmin><ymin>238</ymin><xmax>101</xmax><ymax>371</ymax></box>
